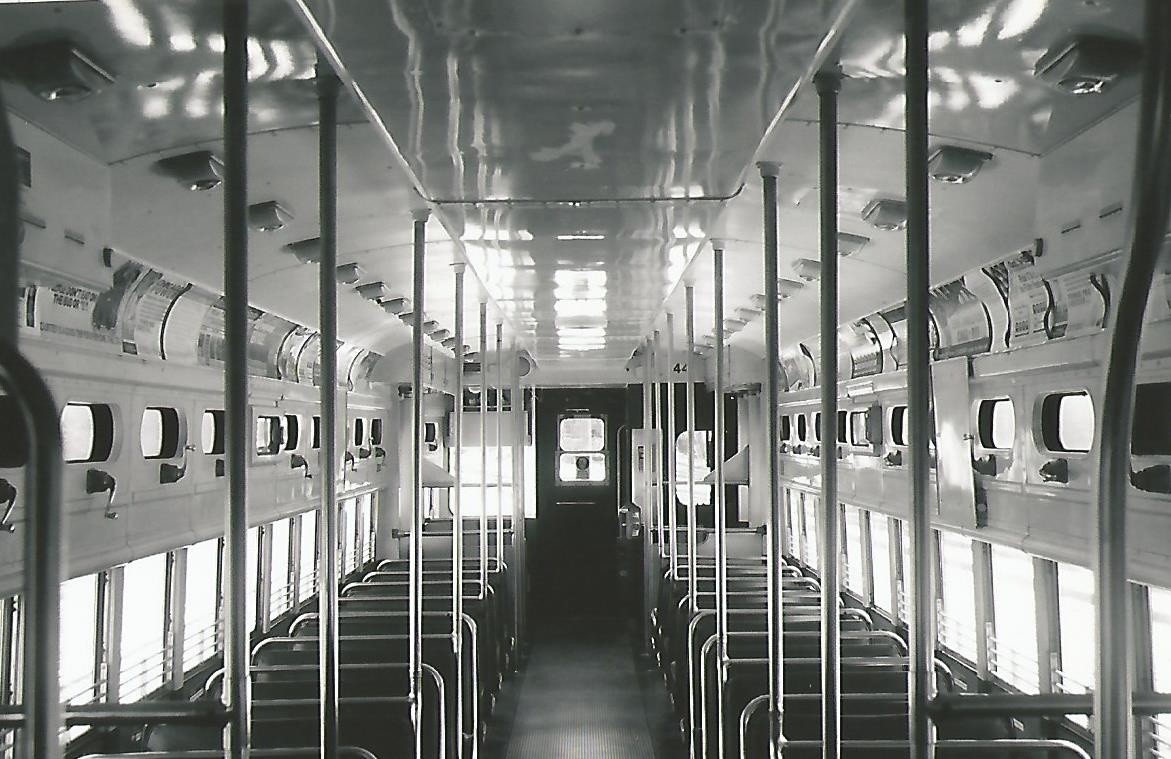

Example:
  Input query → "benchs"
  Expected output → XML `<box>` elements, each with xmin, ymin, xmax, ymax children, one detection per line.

<box><xmin>649</xmin><ymin>558</ymin><xmax>1091</xmax><ymax>758</ymax></box>
<box><xmin>80</xmin><ymin>549</ymin><xmax>512</xmax><ymax>759</ymax></box>
